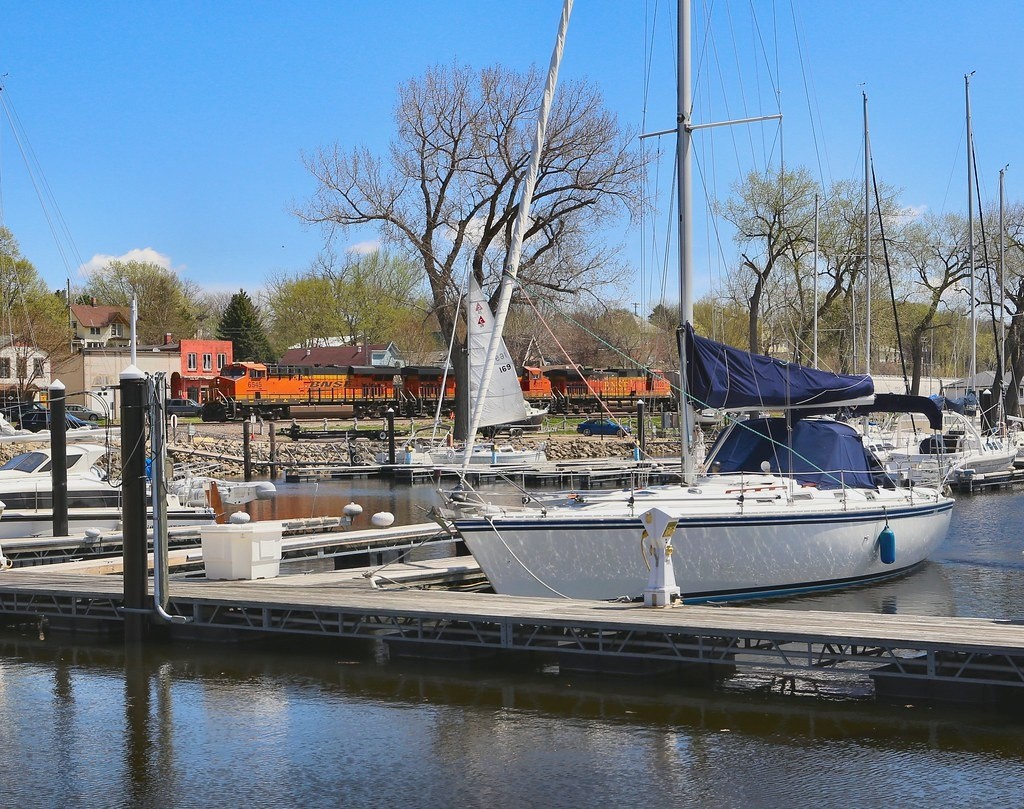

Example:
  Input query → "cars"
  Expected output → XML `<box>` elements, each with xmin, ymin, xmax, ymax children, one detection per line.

<box><xmin>577</xmin><ymin>417</ymin><xmax>632</xmax><ymax>437</ymax></box>
<box><xmin>166</xmin><ymin>399</ymin><xmax>203</xmax><ymax>418</ymax></box>
<box><xmin>14</xmin><ymin>403</ymin><xmax>99</xmax><ymax>433</ymax></box>
<box><xmin>693</xmin><ymin>408</ymin><xmax>721</xmax><ymax>425</ymax></box>
<box><xmin>65</xmin><ymin>403</ymin><xmax>103</xmax><ymax>421</ymax></box>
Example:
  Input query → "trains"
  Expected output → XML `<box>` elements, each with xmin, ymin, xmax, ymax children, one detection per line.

<box><xmin>202</xmin><ymin>361</ymin><xmax>679</xmax><ymax>423</ymax></box>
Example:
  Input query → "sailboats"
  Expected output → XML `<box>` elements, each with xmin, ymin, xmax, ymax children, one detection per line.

<box><xmin>374</xmin><ymin>0</ymin><xmax>1024</xmax><ymax>603</ymax></box>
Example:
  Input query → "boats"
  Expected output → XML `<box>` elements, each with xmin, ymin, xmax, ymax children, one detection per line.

<box><xmin>0</xmin><ymin>443</ymin><xmax>277</xmax><ymax>543</ymax></box>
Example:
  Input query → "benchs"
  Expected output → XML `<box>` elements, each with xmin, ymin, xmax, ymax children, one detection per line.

<box><xmin>288</xmin><ymin>403</ymin><xmax>354</xmax><ymax>420</ymax></box>
